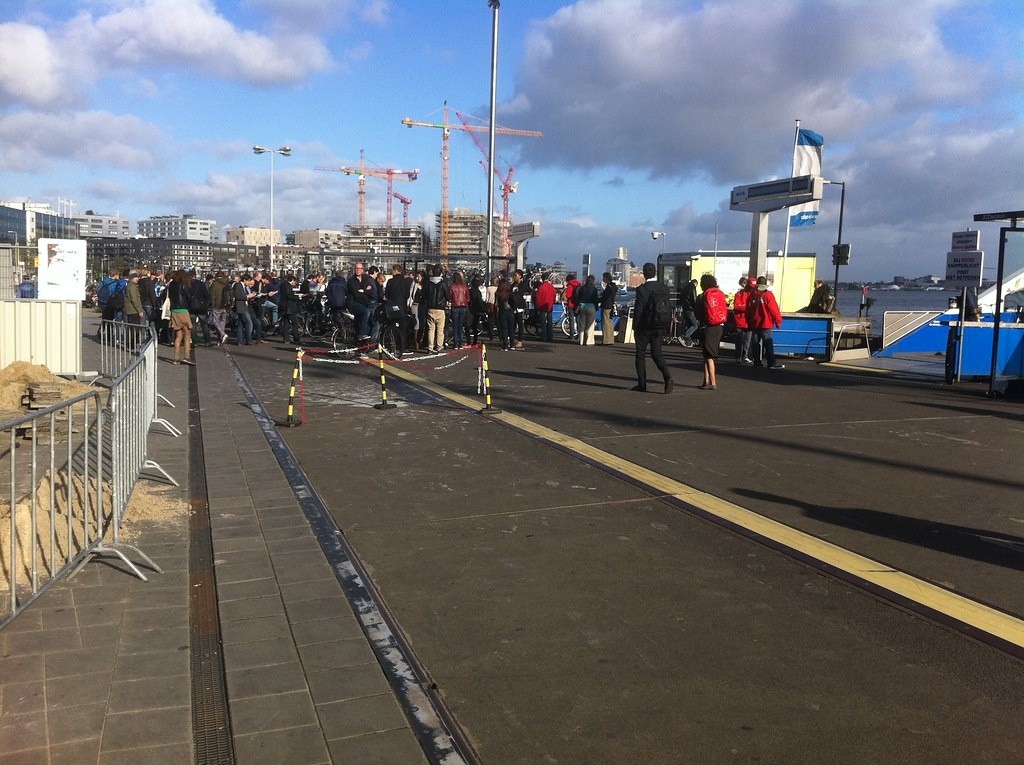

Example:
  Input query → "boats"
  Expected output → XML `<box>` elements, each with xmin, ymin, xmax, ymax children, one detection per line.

<box><xmin>600</xmin><ymin>264</ymin><xmax>628</xmax><ymax>295</ymax></box>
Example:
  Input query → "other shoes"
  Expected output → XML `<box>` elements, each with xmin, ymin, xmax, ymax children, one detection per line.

<box><xmin>770</xmin><ymin>364</ymin><xmax>786</xmax><ymax>369</ymax></box>
<box><xmin>698</xmin><ymin>384</ymin><xmax>717</xmax><ymax>390</ymax></box>
<box><xmin>632</xmin><ymin>385</ymin><xmax>646</xmax><ymax>392</ymax></box>
<box><xmin>160</xmin><ymin>335</ymin><xmax>305</xmax><ymax>366</ymax></box>
<box><xmin>737</xmin><ymin>357</ymin><xmax>754</xmax><ymax>366</ymax></box>
<box><xmin>665</xmin><ymin>376</ymin><xmax>673</xmax><ymax>393</ymax></box>
<box><xmin>428</xmin><ymin>335</ymin><xmax>579</xmax><ymax>355</ymax></box>
<box><xmin>402</xmin><ymin>351</ymin><xmax>414</xmax><ymax>355</ymax></box>
<box><xmin>754</xmin><ymin>361</ymin><xmax>765</xmax><ymax>368</ymax></box>
<box><xmin>358</xmin><ymin>335</ymin><xmax>371</xmax><ymax>341</ymax></box>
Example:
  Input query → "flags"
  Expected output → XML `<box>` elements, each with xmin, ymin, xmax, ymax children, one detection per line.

<box><xmin>789</xmin><ymin>128</ymin><xmax>823</xmax><ymax>228</ymax></box>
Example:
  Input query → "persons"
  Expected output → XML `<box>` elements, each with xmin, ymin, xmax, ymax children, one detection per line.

<box><xmin>600</xmin><ymin>272</ymin><xmax>618</xmax><ymax>346</ymax></box>
<box><xmin>575</xmin><ymin>276</ymin><xmax>599</xmax><ymax>346</ymax></box>
<box><xmin>565</xmin><ymin>274</ymin><xmax>581</xmax><ymax>339</ymax></box>
<box><xmin>678</xmin><ymin>279</ymin><xmax>698</xmax><ymax>347</ymax></box>
<box><xmin>958</xmin><ymin>286</ymin><xmax>980</xmax><ymax>322</ymax></box>
<box><xmin>346</xmin><ymin>263</ymin><xmax>375</xmax><ymax>359</ymax></box>
<box><xmin>463</xmin><ymin>268</ymin><xmax>556</xmax><ymax>352</ymax></box>
<box><xmin>161</xmin><ymin>271</ymin><xmax>197</xmax><ymax>366</ymax></box>
<box><xmin>16</xmin><ymin>273</ymin><xmax>38</xmax><ymax>298</ymax></box>
<box><xmin>278</xmin><ymin>275</ymin><xmax>306</xmax><ymax>346</ymax></box>
<box><xmin>807</xmin><ymin>280</ymin><xmax>829</xmax><ymax>313</ymax></box>
<box><xmin>369</xmin><ymin>264</ymin><xmax>469</xmax><ymax>356</ymax></box>
<box><xmin>747</xmin><ymin>276</ymin><xmax>786</xmax><ymax>369</ymax></box>
<box><xmin>96</xmin><ymin>267</ymin><xmax>174</xmax><ymax>353</ymax></box>
<box><xmin>632</xmin><ymin>263</ymin><xmax>673</xmax><ymax>393</ymax></box>
<box><xmin>181</xmin><ymin>265</ymin><xmax>281</xmax><ymax>348</ymax></box>
<box><xmin>299</xmin><ymin>269</ymin><xmax>347</xmax><ymax>327</ymax></box>
<box><xmin>696</xmin><ymin>275</ymin><xmax>729</xmax><ymax>390</ymax></box>
<box><xmin>734</xmin><ymin>277</ymin><xmax>758</xmax><ymax>363</ymax></box>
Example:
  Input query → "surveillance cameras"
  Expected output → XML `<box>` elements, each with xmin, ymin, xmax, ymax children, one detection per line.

<box><xmin>651</xmin><ymin>232</ymin><xmax>659</xmax><ymax>240</ymax></box>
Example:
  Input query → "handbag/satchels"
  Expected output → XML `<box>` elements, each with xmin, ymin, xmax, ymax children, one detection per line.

<box><xmin>610</xmin><ymin>303</ymin><xmax>618</xmax><ymax>319</ymax></box>
<box><xmin>161</xmin><ymin>289</ymin><xmax>171</xmax><ymax>320</ymax></box>
<box><xmin>189</xmin><ymin>295</ymin><xmax>209</xmax><ymax>314</ymax></box>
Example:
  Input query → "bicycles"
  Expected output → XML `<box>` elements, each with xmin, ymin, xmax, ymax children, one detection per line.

<box><xmin>265</xmin><ymin>292</ymin><xmax>526</xmax><ymax>360</ymax></box>
<box><xmin>526</xmin><ymin>290</ymin><xmax>579</xmax><ymax>337</ymax></box>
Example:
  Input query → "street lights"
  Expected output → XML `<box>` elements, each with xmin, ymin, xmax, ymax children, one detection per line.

<box><xmin>253</xmin><ymin>146</ymin><xmax>291</xmax><ymax>275</ymax></box>
<box><xmin>8</xmin><ymin>231</ymin><xmax>18</xmax><ymax>275</ymax></box>
<box><xmin>102</xmin><ymin>255</ymin><xmax>110</xmax><ymax>282</ymax></box>
<box><xmin>823</xmin><ymin>181</ymin><xmax>845</xmax><ymax>307</ymax></box>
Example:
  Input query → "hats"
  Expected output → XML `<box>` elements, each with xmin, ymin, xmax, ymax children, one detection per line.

<box><xmin>129</xmin><ymin>270</ymin><xmax>139</xmax><ymax>280</ymax></box>
<box><xmin>748</xmin><ymin>278</ymin><xmax>757</xmax><ymax>288</ymax></box>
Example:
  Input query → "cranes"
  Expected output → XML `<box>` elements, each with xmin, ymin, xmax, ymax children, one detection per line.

<box><xmin>314</xmin><ymin>149</ymin><xmax>420</xmax><ymax>235</ymax></box>
<box><xmin>456</xmin><ymin>112</ymin><xmax>519</xmax><ymax>266</ymax></box>
<box><xmin>401</xmin><ymin>100</ymin><xmax>544</xmax><ymax>268</ymax></box>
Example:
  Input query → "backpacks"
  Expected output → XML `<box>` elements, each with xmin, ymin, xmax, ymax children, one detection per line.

<box><xmin>221</xmin><ymin>283</ymin><xmax>236</xmax><ymax>309</ymax></box>
<box><xmin>97</xmin><ymin>281</ymin><xmax>116</xmax><ymax>306</ymax></box>
<box><xmin>703</xmin><ymin>287</ymin><xmax>729</xmax><ymax>326</ymax></box>
<box><xmin>567</xmin><ymin>283</ymin><xmax>581</xmax><ymax>304</ymax></box>
<box><xmin>643</xmin><ymin>283</ymin><xmax>672</xmax><ymax>329</ymax></box>
<box><xmin>110</xmin><ymin>283</ymin><xmax>125</xmax><ymax>311</ymax></box>
